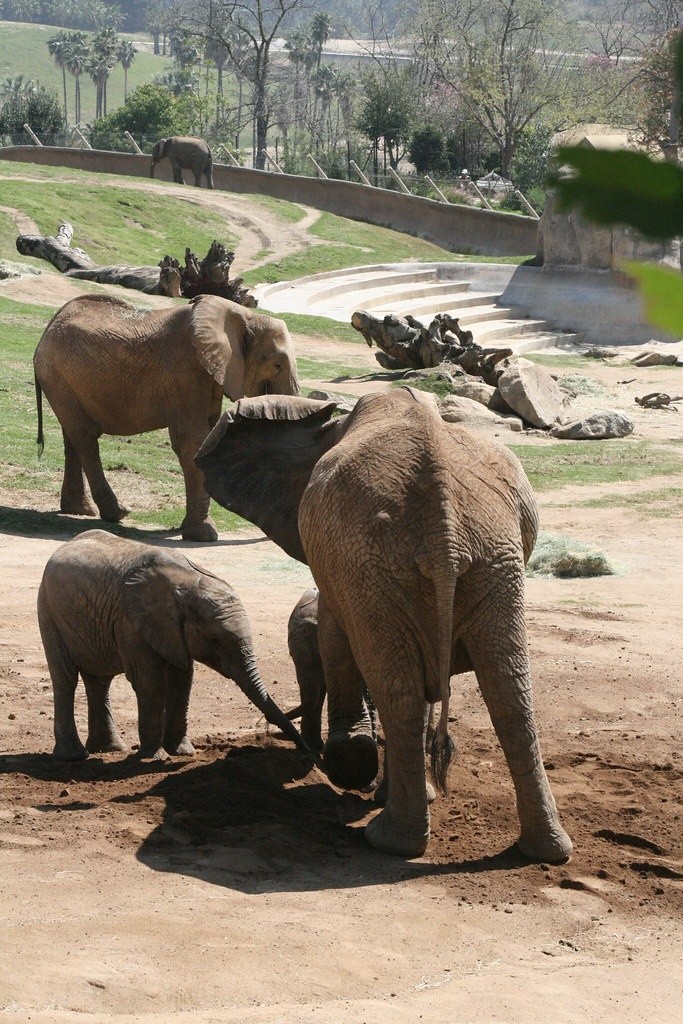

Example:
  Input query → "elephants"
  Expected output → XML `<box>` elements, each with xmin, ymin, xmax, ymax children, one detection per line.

<box><xmin>33</xmin><ymin>293</ymin><xmax>301</xmax><ymax>542</ymax></box>
<box><xmin>150</xmin><ymin>136</ymin><xmax>214</xmax><ymax>191</ymax></box>
<box><xmin>35</xmin><ymin>385</ymin><xmax>573</xmax><ymax>867</ymax></box>
